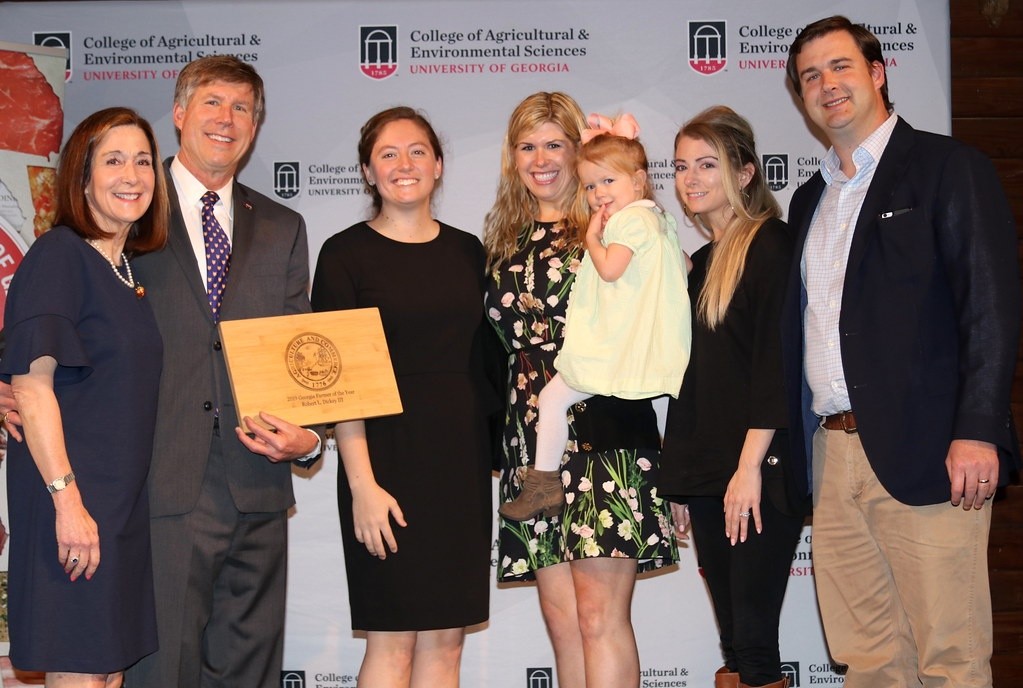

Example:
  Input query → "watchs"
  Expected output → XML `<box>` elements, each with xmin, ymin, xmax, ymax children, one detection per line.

<box><xmin>47</xmin><ymin>472</ymin><xmax>75</xmax><ymax>493</ymax></box>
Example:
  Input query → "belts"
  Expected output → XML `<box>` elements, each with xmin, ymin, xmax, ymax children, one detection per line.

<box><xmin>822</xmin><ymin>411</ymin><xmax>857</xmax><ymax>434</ymax></box>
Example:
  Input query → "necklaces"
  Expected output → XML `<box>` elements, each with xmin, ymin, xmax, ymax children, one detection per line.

<box><xmin>91</xmin><ymin>239</ymin><xmax>135</xmax><ymax>289</ymax></box>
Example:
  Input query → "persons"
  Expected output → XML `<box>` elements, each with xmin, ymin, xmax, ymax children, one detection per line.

<box><xmin>656</xmin><ymin>105</ymin><xmax>809</xmax><ymax>687</ymax></box>
<box><xmin>483</xmin><ymin>92</ymin><xmax>679</xmax><ymax>688</ymax></box>
<box><xmin>499</xmin><ymin>113</ymin><xmax>694</xmax><ymax>521</ymax></box>
<box><xmin>0</xmin><ymin>54</ymin><xmax>325</xmax><ymax>688</ymax></box>
<box><xmin>1</xmin><ymin>107</ymin><xmax>168</xmax><ymax>688</ymax></box>
<box><xmin>787</xmin><ymin>17</ymin><xmax>1016</xmax><ymax>688</ymax></box>
<box><xmin>311</xmin><ymin>108</ymin><xmax>508</xmax><ymax>688</ymax></box>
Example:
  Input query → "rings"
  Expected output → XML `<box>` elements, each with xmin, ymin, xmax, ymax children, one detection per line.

<box><xmin>978</xmin><ymin>479</ymin><xmax>989</xmax><ymax>483</ymax></box>
<box><xmin>1</xmin><ymin>410</ymin><xmax>19</xmax><ymax>420</ymax></box>
<box><xmin>740</xmin><ymin>512</ymin><xmax>750</xmax><ymax>518</ymax></box>
<box><xmin>68</xmin><ymin>555</ymin><xmax>79</xmax><ymax>564</ymax></box>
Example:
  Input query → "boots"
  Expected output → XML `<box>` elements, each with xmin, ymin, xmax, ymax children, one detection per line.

<box><xmin>737</xmin><ymin>676</ymin><xmax>789</xmax><ymax>688</ymax></box>
<box><xmin>715</xmin><ymin>666</ymin><xmax>740</xmax><ymax>688</ymax></box>
<box><xmin>497</xmin><ymin>465</ymin><xmax>565</xmax><ymax>522</ymax></box>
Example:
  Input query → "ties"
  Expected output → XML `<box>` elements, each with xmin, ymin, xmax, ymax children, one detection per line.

<box><xmin>199</xmin><ymin>191</ymin><xmax>232</xmax><ymax>419</ymax></box>
